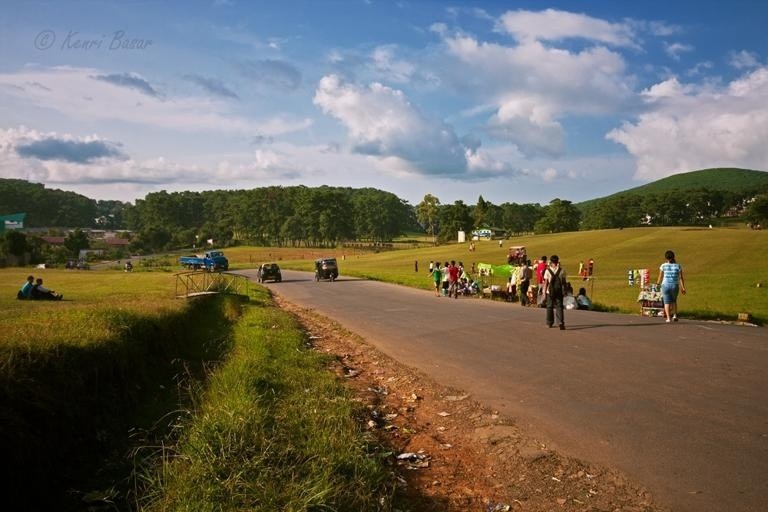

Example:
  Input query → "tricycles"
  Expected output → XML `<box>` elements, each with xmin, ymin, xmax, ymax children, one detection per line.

<box><xmin>315</xmin><ymin>258</ymin><xmax>338</xmax><ymax>282</ymax></box>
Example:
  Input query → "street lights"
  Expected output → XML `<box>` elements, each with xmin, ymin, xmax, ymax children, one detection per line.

<box><xmin>432</xmin><ymin>223</ymin><xmax>438</xmax><ymax>246</ymax></box>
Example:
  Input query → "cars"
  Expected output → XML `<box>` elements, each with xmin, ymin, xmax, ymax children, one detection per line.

<box><xmin>65</xmin><ymin>260</ymin><xmax>77</xmax><ymax>269</ymax></box>
<box><xmin>77</xmin><ymin>262</ymin><xmax>89</xmax><ymax>269</ymax></box>
<box><xmin>37</xmin><ymin>263</ymin><xmax>48</xmax><ymax>269</ymax></box>
<box><xmin>256</xmin><ymin>263</ymin><xmax>282</xmax><ymax>283</ymax></box>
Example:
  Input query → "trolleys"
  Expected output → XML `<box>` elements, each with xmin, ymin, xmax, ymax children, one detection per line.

<box><xmin>476</xmin><ymin>262</ymin><xmax>514</xmax><ymax>303</ymax></box>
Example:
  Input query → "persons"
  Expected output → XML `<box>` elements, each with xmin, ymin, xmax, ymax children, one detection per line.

<box><xmin>327</xmin><ymin>272</ymin><xmax>333</xmax><ymax>287</ymax></box>
<box><xmin>29</xmin><ymin>278</ymin><xmax>62</xmax><ymax>301</ymax></box>
<box><xmin>541</xmin><ymin>255</ymin><xmax>567</xmax><ymax>331</ymax></box>
<box><xmin>312</xmin><ymin>267</ymin><xmax>320</xmax><ymax>285</ymax></box>
<box><xmin>20</xmin><ymin>276</ymin><xmax>34</xmax><ymax>298</ymax></box>
<box><xmin>654</xmin><ymin>250</ymin><xmax>686</xmax><ymax>324</ymax></box>
<box><xmin>413</xmin><ymin>237</ymin><xmax>593</xmax><ymax>310</ymax></box>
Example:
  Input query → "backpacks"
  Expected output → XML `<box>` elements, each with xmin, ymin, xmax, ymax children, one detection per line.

<box><xmin>548</xmin><ymin>268</ymin><xmax>562</xmax><ymax>300</ymax></box>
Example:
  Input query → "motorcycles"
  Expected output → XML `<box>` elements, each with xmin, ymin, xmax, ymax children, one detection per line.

<box><xmin>123</xmin><ymin>265</ymin><xmax>133</xmax><ymax>273</ymax></box>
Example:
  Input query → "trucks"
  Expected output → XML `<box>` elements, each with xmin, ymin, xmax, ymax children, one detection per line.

<box><xmin>179</xmin><ymin>250</ymin><xmax>229</xmax><ymax>272</ymax></box>
